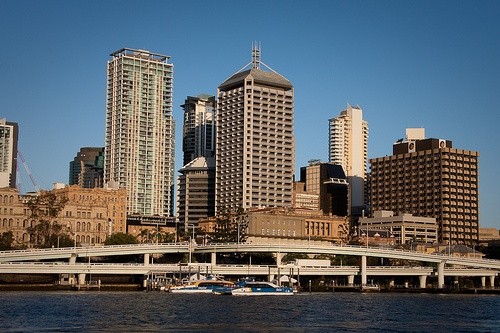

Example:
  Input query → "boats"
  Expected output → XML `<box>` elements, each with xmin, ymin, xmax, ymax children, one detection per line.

<box><xmin>167</xmin><ymin>276</ymin><xmax>241</xmax><ymax>294</ymax></box>
<box><xmin>211</xmin><ymin>278</ymin><xmax>296</xmax><ymax>297</ymax></box>
